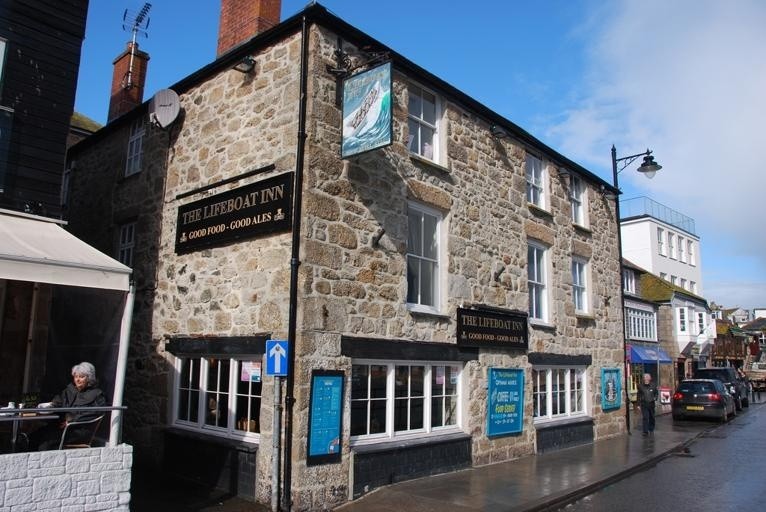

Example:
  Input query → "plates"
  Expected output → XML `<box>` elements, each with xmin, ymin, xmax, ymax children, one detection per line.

<box><xmin>37</xmin><ymin>412</ymin><xmax>55</xmax><ymax>414</ymax></box>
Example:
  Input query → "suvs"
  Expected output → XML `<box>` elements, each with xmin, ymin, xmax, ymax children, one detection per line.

<box><xmin>692</xmin><ymin>366</ymin><xmax>750</xmax><ymax>412</ymax></box>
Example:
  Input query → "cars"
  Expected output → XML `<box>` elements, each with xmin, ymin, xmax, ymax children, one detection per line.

<box><xmin>671</xmin><ymin>378</ymin><xmax>738</xmax><ymax>422</ymax></box>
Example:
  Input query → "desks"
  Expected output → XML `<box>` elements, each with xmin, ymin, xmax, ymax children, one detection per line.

<box><xmin>0</xmin><ymin>413</ymin><xmax>59</xmax><ymax>452</ymax></box>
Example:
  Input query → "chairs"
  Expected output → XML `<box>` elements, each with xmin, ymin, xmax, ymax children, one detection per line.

<box><xmin>60</xmin><ymin>416</ymin><xmax>103</xmax><ymax>449</ymax></box>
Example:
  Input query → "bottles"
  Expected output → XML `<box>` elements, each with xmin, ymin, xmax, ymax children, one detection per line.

<box><xmin>8</xmin><ymin>401</ymin><xmax>16</xmax><ymax>415</ymax></box>
<box><xmin>18</xmin><ymin>403</ymin><xmax>26</xmax><ymax>415</ymax></box>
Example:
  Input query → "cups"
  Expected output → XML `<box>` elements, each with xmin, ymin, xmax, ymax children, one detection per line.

<box><xmin>38</xmin><ymin>403</ymin><xmax>52</xmax><ymax>412</ymax></box>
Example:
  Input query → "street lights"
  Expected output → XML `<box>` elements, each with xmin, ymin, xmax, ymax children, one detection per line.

<box><xmin>610</xmin><ymin>145</ymin><xmax>663</xmax><ymax>432</ymax></box>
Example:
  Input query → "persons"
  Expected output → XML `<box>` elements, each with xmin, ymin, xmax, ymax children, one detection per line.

<box><xmin>636</xmin><ymin>372</ymin><xmax>660</xmax><ymax>438</ymax></box>
<box><xmin>29</xmin><ymin>359</ymin><xmax>106</xmax><ymax>453</ymax></box>
<box><xmin>741</xmin><ymin>372</ymin><xmax>749</xmax><ymax>386</ymax></box>
